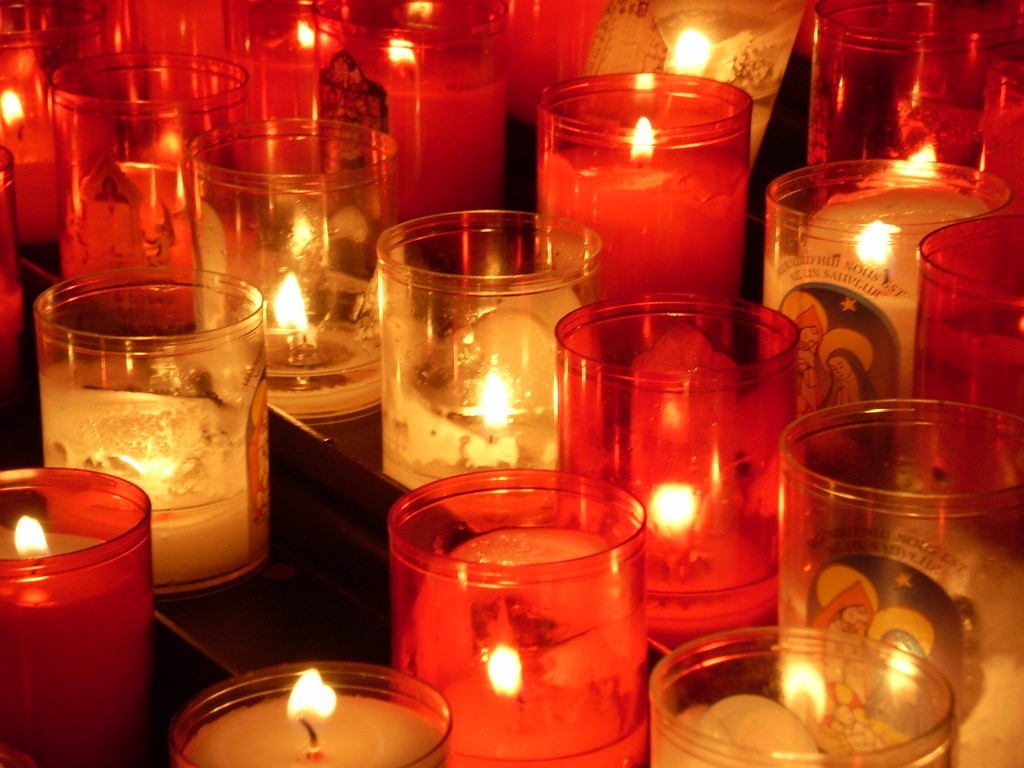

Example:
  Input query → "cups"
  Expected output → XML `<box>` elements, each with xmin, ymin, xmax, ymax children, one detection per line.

<box><xmin>764</xmin><ymin>159</ymin><xmax>1014</xmax><ymax>423</ymax></box>
<box><xmin>36</xmin><ymin>269</ymin><xmax>270</xmax><ymax>593</ymax></box>
<box><xmin>314</xmin><ymin>0</ymin><xmax>510</xmax><ymax>227</ymax></box>
<box><xmin>648</xmin><ymin>628</ymin><xmax>956</xmax><ymax>768</ymax></box>
<box><xmin>47</xmin><ymin>53</ymin><xmax>250</xmax><ymax>333</ymax></box>
<box><xmin>0</xmin><ymin>146</ymin><xmax>22</xmax><ymax>382</ymax></box>
<box><xmin>0</xmin><ymin>468</ymin><xmax>153</xmax><ymax>768</ymax></box>
<box><xmin>0</xmin><ymin>0</ymin><xmax>109</xmax><ymax>245</ymax></box>
<box><xmin>376</xmin><ymin>212</ymin><xmax>603</xmax><ymax>492</ymax></box>
<box><xmin>194</xmin><ymin>118</ymin><xmax>399</xmax><ymax>424</ymax></box>
<box><xmin>225</xmin><ymin>0</ymin><xmax>315</xmax><ymax>171</ymax></box>
<box><xmin>510</xmin><ymin>0</ymin><xmax>807</xmax><ymax>168</ymax></box>
<box><xmin>807</xmin><ymin>0</ymin><xmax>1024</xmax><ymax>170</ymax></box>
<box><xmin>556</xmin><ymin>294</ymin><xmax>802</xmax><ymax>653</ymax></box>
<box><xmin>539</xmin><ymin>71</ymin><xmax>752</xmax><ymax>295</ymax></box>
<box><xmin>978</xmin><ymin>53</ymin><xmax>1024</xmax><ymax>212</ymax></box>
<box><xmin>776</xmin><ymin>401</ymin><xmax>1024</xmax><ymax>768</ymax></box>
<box><xmin>387</xmin><ymin>470</ymin><xmax>646</xmax><ymax>768</ymax></box>
<box><xmin>913</xmin><ymin>212</ymin><xmax>1024</xmax><ymax>416</ymax></box>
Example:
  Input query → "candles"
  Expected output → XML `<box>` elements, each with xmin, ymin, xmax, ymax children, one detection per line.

<box><xmin>0</xmin><ymin>0</ymin><xmax>1024</xmax><ymax>768</ymax></box>
